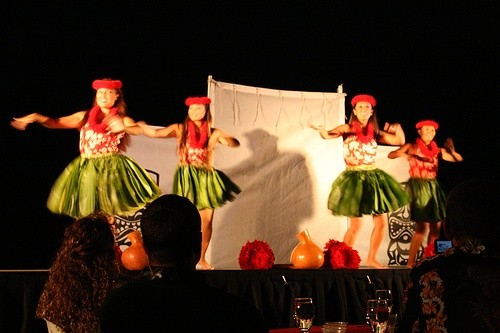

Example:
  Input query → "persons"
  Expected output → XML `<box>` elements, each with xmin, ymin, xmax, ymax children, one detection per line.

<box><xmin>36</xmin><ymin>210</ymin><xmax>130</xmax><ymax>333</ymax></box>
<box><xmin>393</xmin><ymin>179</ymin><xmax>500</xmax><ymax>333</ymax></box>
<box><xmin>387</xmin><ymin>120</ymin><xmax>463</xmax><ymax>269</ymax></box>
<box><xmin>12</xmin><ymin>79</ymin><xmax>163</xmax><ymax>265</ymax></box>
<box><xmin>306</xmin><ymin>94</ymin><xmax>405</xmax><ymax>269</ymax></box>
<box><xmin>98</xmin><ymin>194</ymin><xmax>270</xmax><ymax>333</ymax></box>
<box><xmin>127</xmin><ymin>97</ymin><xmax>240</xmax><ymax>270</ymax></box>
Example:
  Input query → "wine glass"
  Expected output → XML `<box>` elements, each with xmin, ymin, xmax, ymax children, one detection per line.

<box><xmin>293</xmin><ymin>298</ymin><xmax>314</xmax><ymax>332</ymax></box>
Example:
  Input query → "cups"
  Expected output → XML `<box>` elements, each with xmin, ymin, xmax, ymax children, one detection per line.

<box><xmin>368</xmin><ymin>300</ymin><xmax>389</xmax><ymax>333</ymax></box>
<box><xmin>376</xmin><ymin>290</ymin><xmax>392</xmax><ymax>314</ymax></box>
<box><xmin>321</xmin><ymin>322</ymin><xmax>348</xmax><ymax>333</ymax></box>
<box><xmin>388</xmin><ymin>315</ymin><xmax>397</xmax><ymax>333</ymax></box>
